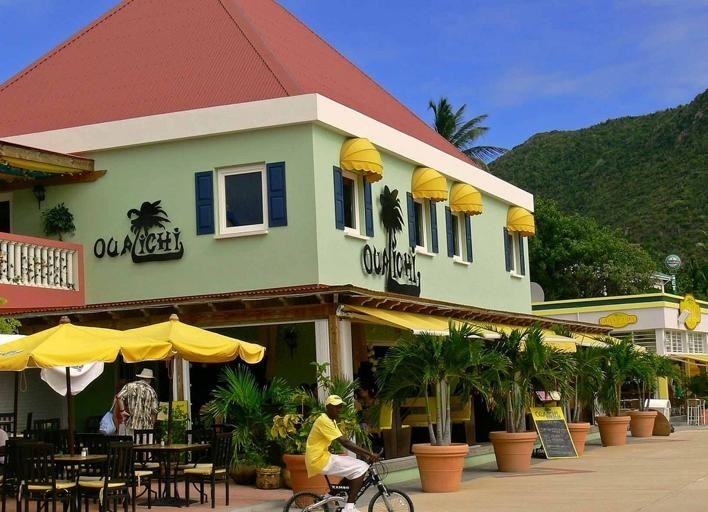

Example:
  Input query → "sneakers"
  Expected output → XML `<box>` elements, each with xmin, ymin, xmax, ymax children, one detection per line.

<box><xmin>325</xmin><ymin>492</ymin><xmax>345</xmax><ymax>503</ymax></box>
<box><xmin>343</xmin><ymin>506</ymin><xmax>363</xmax><ymax>512</ymax></box>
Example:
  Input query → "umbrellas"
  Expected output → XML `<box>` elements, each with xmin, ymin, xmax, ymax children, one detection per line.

<box><xmin>0</xmin><ymin>317</ymin><xmax>171</xmax><ymax>512</ymax></box>
<box><xmin>121</xmin><ymin>313</ymin><xmax>269</xmax><ymax>498</ymax></box>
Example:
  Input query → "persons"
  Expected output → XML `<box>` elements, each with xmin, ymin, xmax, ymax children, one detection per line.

<box><xmin>305</xmin><ymin>395</ymin><xmax>377</xmax><ymax>512</ymax></box>
<box><xmin>115</xmin><ymin>366</ymin><xmax>160</xmax><ymax>483</ymax></box>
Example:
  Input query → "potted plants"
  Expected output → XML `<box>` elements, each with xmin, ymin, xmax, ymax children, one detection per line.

<box><xmin>202</xmin><ymin>359</ymin><xmax>368</xmax><ymax>508</ymax></box>
<box><xmin>687</xmin><ymin>372</ymin><xmax>707</xmax><ymax>426</ymax></box>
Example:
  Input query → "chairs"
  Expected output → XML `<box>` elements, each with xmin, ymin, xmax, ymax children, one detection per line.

<box><xmin>0</xmin><ymin>401</ymin><xmax>241</xmax><ymax>512</ymax></box>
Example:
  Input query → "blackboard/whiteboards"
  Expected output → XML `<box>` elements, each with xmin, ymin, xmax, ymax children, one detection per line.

<box><xmin>536</xmin><ymin>420</ymin><xmax>580</xmax><ymax>459</ymax></box>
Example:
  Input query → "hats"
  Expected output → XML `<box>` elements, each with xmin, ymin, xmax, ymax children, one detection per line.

<box><xmin>136</xmin><ymin>368</ymin><xmax>156</xmax><ymax>379</ymax></box>
<box><xmin>326</xmin><ymin>394</ymin><xmax>346</xmax><ymax>409</ymax></box>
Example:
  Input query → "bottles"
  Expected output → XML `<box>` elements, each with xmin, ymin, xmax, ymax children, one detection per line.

<box><xmin>81</xmin><ymin>447</ymin><xmax>88</xmax><ymax>457</ymax></box>
<box><xmin>118</xmin><ymin>420</ymin><xmax>132</xmax><ymax>436</ymax></box>
<box><xmin>160</xmin><ymin>438</ymin><xmax>165</xmax><ymax>446</ymax></box>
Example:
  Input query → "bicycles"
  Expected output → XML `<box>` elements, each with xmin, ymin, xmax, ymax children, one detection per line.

<box><xmin>282</xmin><ymin>448</ymin><xmax>416</xmax><ymax>512</ymax></box>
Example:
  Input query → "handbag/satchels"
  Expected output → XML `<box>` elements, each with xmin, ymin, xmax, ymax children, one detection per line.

<box><xmin>99</xmin><ymin>412</ymin><xmax>116</xmax><ymax>435</ymax></box>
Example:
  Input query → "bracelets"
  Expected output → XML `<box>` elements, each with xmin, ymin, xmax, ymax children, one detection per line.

<box><xmin>119</xmin><ymin>409</ymin><xmax>124</xmax><ymax>412</ymax></box>
